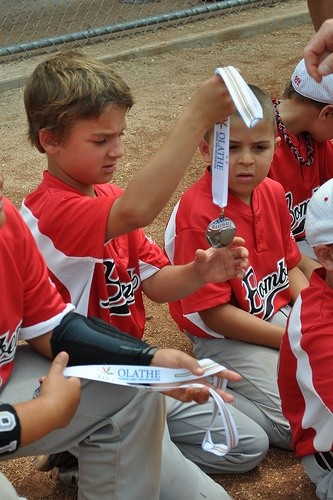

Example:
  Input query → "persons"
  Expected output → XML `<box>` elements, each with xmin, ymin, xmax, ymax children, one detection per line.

<box><xmin>163</xmin><ymin>15</ymin><xmax>333</xmax><ymax>500</ymax></box>
<box><xmin>0</xmin><ymin>50</ymin><xmax>249</xmax><ymax>500</ymax></box>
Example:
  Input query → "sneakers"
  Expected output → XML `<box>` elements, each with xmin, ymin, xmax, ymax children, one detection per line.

<box><xmin>35</xmin><ymin>451</ymin><xmax>81</xmax><ymax>474</ymax></box>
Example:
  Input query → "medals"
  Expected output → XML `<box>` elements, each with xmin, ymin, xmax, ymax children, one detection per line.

<box><xmin>205</xmin><ymin>215</ymin><xmax>236</xmax><ymax>248</ymax></box>
<box><xmin>32</xmin><ymin>385</ymin><xmax>40</xmax><ymax>398</ymax></box>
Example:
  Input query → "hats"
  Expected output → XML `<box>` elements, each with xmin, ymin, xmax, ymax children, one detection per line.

<box><xmin>290</xmin><ymin>58</ymin><xmax>333</xmax><ymax>104</ymax></box>
<box><xmin>304</xmin><ymin>178</ymin><xmax>333</xmax><ymax>247</ymax></box>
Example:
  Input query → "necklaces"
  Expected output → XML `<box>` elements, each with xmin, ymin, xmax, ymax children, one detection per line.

<box><xmin>272</xmin><ymin>99</ymin><xmax>314</xmax><ymax>167</ymax></box>
